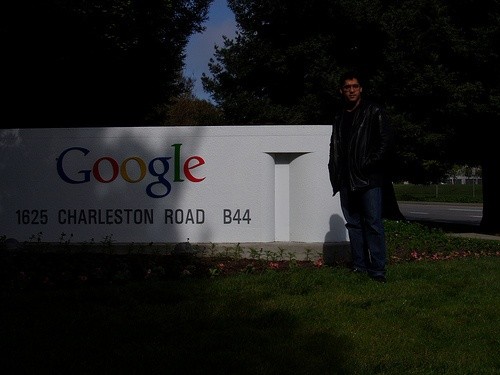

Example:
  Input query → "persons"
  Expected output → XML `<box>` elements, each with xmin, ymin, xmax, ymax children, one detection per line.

<box><xmin>328</xmin><ymin>70</ymin><xmax>405</xmax><ymax>284</ymax></box>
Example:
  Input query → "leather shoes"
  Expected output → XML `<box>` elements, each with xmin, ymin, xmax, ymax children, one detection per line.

<box><xmin>347</xmin><ymin>267</ymin><xmax>360</xmax><ymax>273</ymax></box>
<box><xmin>371</xmin><ymin>273</ymin><xmax>387</xmax><ymax>283</ymax></box>
